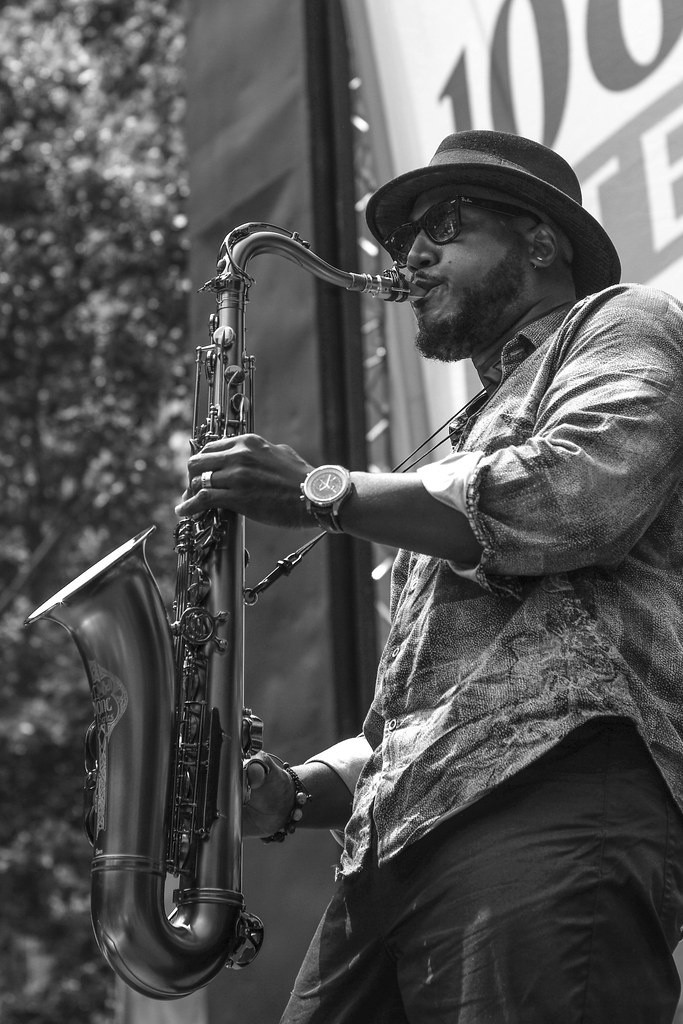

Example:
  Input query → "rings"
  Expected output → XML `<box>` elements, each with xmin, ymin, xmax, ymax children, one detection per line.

<box><xmin>201</xmin><ymin>469</ymin><xmax>214</xmax><ymax>488</ymax></box>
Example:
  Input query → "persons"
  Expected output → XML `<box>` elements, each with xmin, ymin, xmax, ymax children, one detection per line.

<box><xmin>174</xmin><ymin>129</ymin><xmax>683</xmax><ymax>1024</ymax></box>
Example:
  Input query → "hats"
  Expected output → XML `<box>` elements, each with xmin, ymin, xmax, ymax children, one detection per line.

<box><xmin>366</xmin><ymin>130</ymin><xmax>622</xmax><ymax>299</ymax></box>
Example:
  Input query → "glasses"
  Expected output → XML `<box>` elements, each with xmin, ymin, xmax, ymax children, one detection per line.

<box><xmin>382</xmin><ymin>194</ymin><xmax>542</xmax><ymax>268</ymax></box>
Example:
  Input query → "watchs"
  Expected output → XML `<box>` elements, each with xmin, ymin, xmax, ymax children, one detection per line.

<box><xmin>298</xmin><ymin>463</ymin><xmax>352</xmax><ymax>536</ymax></box>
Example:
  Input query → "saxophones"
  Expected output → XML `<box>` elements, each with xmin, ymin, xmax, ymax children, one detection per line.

<box><xmin>18</xmin><ymin>219</ymin><xmax>431</xmax><ymax>1004</ymax></box>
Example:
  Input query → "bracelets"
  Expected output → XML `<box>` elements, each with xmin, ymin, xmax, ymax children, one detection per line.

<box><xmin>260</xmin><ymin>750</ymin><xmax>312</xmax><ymax>846</ymax></box>
